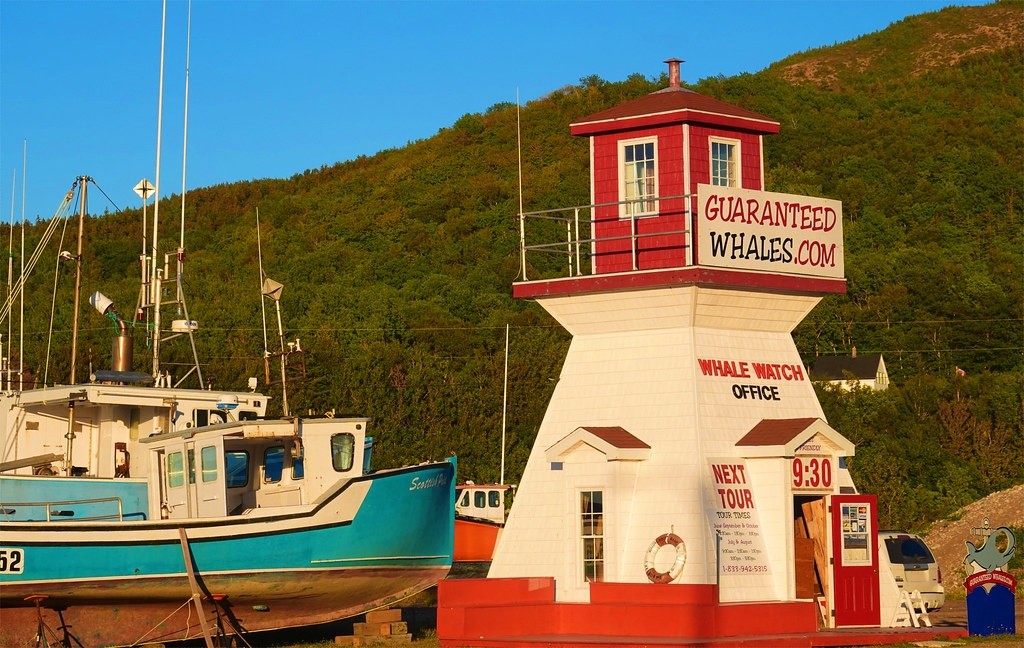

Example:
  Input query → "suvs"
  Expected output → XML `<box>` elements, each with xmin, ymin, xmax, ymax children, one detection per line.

<box><xmin>878</xmin><ymin>529</ymin><xmax>945</xmax><ymax>613</ymax></box>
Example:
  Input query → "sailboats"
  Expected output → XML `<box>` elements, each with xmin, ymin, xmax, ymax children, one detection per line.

<box><xmin>0</xmin><ymin>0</ymin><xmax>517</xmax><ymax>648</ymax></box>
